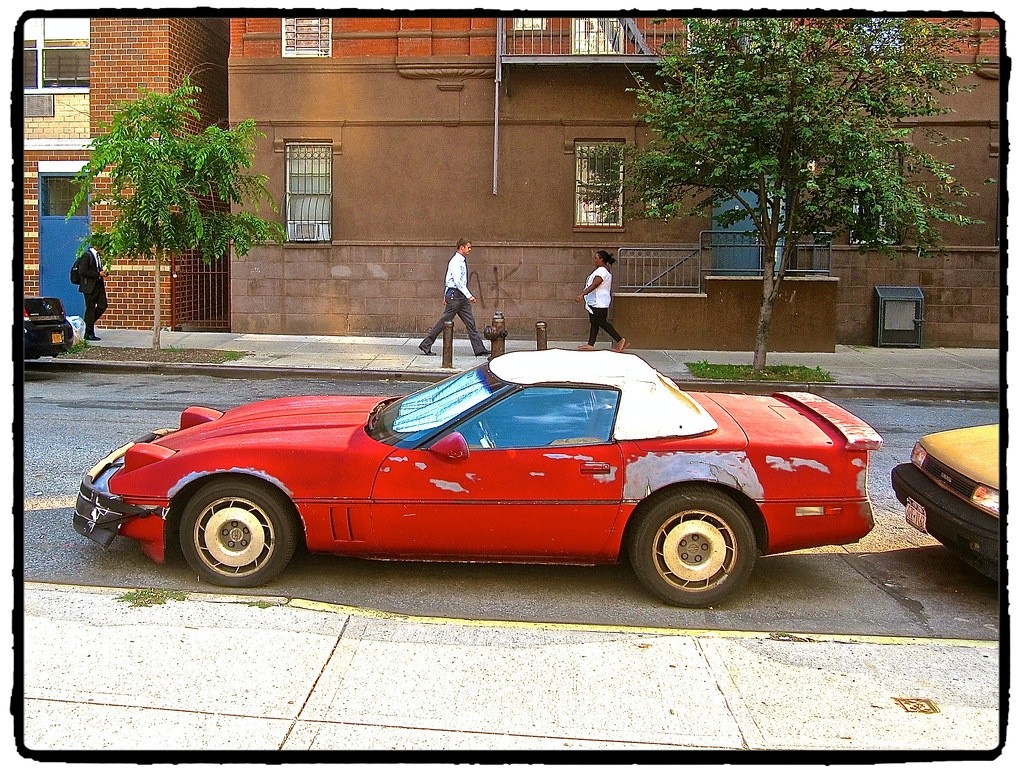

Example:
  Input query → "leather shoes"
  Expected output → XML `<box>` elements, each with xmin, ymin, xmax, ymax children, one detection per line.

<box><xmin>84</xmin><ymin>332</ymin><xmax>101</xmax><ymax>341</ymax></box>
<box><xmin>473</xmin><ymin>349</ymin><xmax>491</xmax><ymax>356</ymax></box>
<box><xmin>419</xmin><ymin>343</ymin><xmax>436</xmax><ymax>355</ymax></box>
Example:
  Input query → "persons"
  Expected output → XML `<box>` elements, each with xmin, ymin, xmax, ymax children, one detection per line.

<box><xmin>79</xmin><ymin>234</ymin><xmax>108</xmax><ymax>341</ymax></box>
<box><xmin>419</xmin><ymin>238</ymin><xmax>491</xmax><ymax>356</ymax></box>
<box><xmin>575</xmin><ymin>251</ymin><xmax>630</xmax><ymax>352</ymax></box>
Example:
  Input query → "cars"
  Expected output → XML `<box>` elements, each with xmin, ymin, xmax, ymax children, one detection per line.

<box><xmin>891</xmin><ymin>423</ymin><xmax>999</xmax><ymax>584</ymax></box>
<box><xmin>108</xmin><ymin>347</ymin><xmax>884</xmax><ymax>608</ymax></box>
<box><xmin>23</xmin><ymin>295</ymin><xmax>75</xmax><ymax>360</ymax></box>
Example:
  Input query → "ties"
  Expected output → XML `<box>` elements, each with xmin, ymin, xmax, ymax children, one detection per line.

<box><xmin>96</xmin><ymin>251</ymin><xmax>101</xmax><ymax>270</ymax></box>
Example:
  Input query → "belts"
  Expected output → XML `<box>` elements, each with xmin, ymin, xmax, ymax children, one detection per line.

<box><xmin>448</xmin><ymin>287</ymin><xmax>460</xmax><ymax>291</ymax></box>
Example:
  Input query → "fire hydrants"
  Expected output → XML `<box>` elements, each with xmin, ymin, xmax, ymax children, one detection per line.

<box><xmin>485</xmin><ymin>310</ymin><xmax>507</xmax><ymax>361</ymax></box>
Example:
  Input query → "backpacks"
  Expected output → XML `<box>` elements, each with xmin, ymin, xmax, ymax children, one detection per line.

<box><xmin>69</xmin><ymin>252</ymin><xmax>93</xmax><ymax>284</ymax></box>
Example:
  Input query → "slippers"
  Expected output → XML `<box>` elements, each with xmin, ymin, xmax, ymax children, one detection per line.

<box><xmin>578</xmin><ymin>343</ymin><xmax>593</xmax><ymax>350</ymax></box>
<box><xmin>618</xmin><ymin>339</ymin><xmax>631</xmax><ymax>353</ymax></box>
